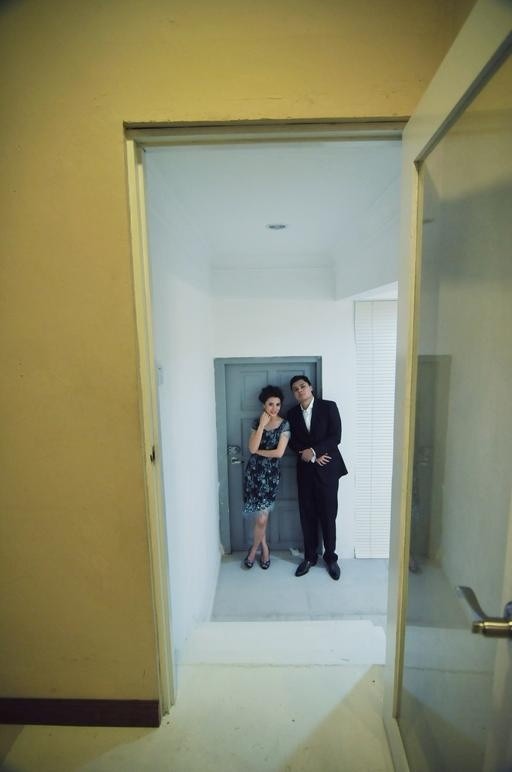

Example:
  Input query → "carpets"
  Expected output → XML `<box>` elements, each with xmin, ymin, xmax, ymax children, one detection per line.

<box><xmin>406</xmin><ymin>554</ymin><xmax>472</xmax><ymax>629</ymax></box>
<box><xmin>210</xmin><ymin>550</ymin><xmax>389</xmax><ymax>621</ymax></box>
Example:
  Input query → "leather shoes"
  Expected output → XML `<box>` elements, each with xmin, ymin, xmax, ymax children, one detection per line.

<box><xmin>244</xmin><ymin>546</ymin><xmax>257</xmax><ymax>568</ymax></box>
<box><xmin>260</xmin><ymin>544</ymin><xmax>271</xmax><ymax>569</ymax></box>
<box><xmin>327</xmin><ymin>561</ymin><xmax>341</xmax><ymax>580</ymax></box>
<box><xmin>295</xmin><ymin>559</ymin><xmax>318</xmax><ymax>577</ymax></box>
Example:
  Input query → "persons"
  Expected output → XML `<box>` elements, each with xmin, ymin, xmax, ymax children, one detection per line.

<box><xmin>284</xmin><ymin>374</ymin><xmax>349</xmax><ymax>582</ymax></box>
<box><xmin>238</xmin><ymin>385</ymin><xmax>293</xmax><ymax>569</ymax></box>
